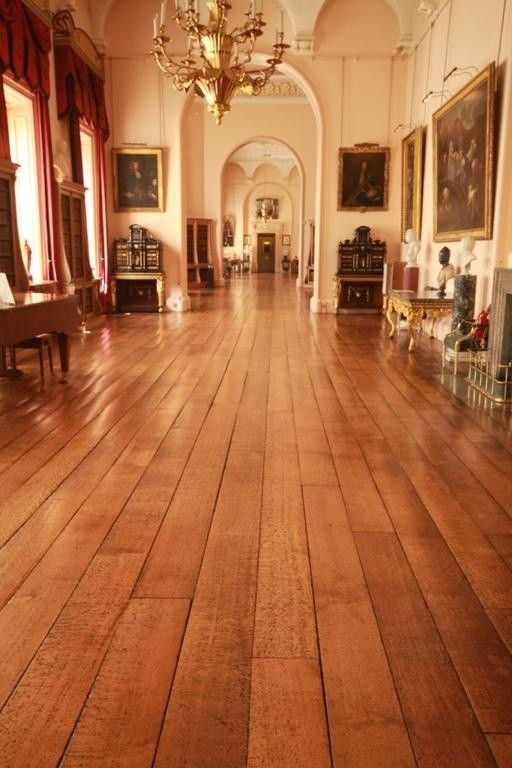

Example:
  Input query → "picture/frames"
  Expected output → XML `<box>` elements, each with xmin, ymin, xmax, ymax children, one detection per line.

<box><xmin>282</xmin><ymin>235</ymin><xmax>289</xmax><ymax>246</ymax></box>
<box><xmin>336</xmin><ymin>147</ymin><xmax>390</xmax><ymax>212</ymax></box>
<box><xmin>432</xmin><ymin>61</ymin><xmax>494</xmax><ymax>243</ymax></box>
<box><xmin>401</xmin><ymin>127</ymin><xmax>424</xmax><ymax>244</ymax></box>
<box><xmin>111</xmin><ymin>147</ymin><xmax>165</xmax><ymax>212</ymax></box>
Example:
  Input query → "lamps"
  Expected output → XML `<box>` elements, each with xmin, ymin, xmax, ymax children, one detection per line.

<box><xmin>150</xmin><ymin>1</ymin><xmax>290</xmax><ymax>127</ymax></box>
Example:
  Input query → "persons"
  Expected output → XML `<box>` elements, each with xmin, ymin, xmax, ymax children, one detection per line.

<box><xmin>346</xmin><ymin>160</ymin><xmax>372</xmax><ymax>189</ymax></box>
<box><xmin>403</xmin><ymin>147</ymin><xmax>417</xmax><ymax>238</ymax></box>
<box><xmin>342</xmin><ymin>183</ymin><xmax>385</xmax><ymax>207</ymax></box>
<box><xmin>457</xmin><ymin>236</ymin><xmax>477</xmax><ymax>276</ymax></box>
<box><xmin>435</xmin><ymin>109</ymin><xmax>485</xmax><ymax>232</ymax></box>
<box><xmin>437</xmin><ymin>246</ymin><xmax>455</xmax><ymax>298</ymax></box>
<box><xmin>404</xmin><ymin>228</ymin><xmax>421</xmax><ymax>267</ymax></box>
<box><xmin>145</xmin><ymin>176</ymin><xmax>159</xmax><ymax>204</ymax></box>
<box><xmin>122</xmin><ymin>157</ymin><xmax>148</xmax><ymax>203</ymax></box>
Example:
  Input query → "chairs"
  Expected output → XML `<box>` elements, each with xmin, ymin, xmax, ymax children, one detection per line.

<box><xmin>4</xmin><ymin>335</ymin><xmax>53</xmax><ymax>388</ymax></box>
<box><xmin>441</xmin><ymin>320</ymin><xmax>488</xmax><ymax>376</ymax></box>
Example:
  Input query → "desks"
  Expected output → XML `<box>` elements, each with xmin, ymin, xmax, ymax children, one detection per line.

<box><xmin>385</xmin><ymin>288</ymin><xmax>455</xmax><ymax>352</ymax></box>
<box><xmin>0</xmin><ymin>291</ymin><xmax>79</xmax><ymax>384</ymax></box>
